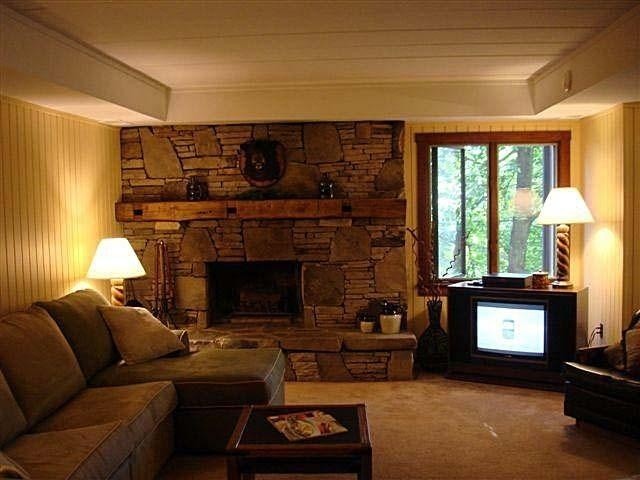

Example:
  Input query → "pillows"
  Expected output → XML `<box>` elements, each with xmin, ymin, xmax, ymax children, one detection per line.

<box><xmin>98</xmin><ymin>307</ymin><xmax>186</xmax><ymax>364</ymax></box>
<box><xmin>622</xmin><ymin>327</ymin><xmax>640</xmax><ymax>376</ymax></box>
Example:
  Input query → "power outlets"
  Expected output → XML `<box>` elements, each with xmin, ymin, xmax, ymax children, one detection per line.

<box><xmin>600</xmin><ymin>323</ymin><xmax>603</xmax><ymax>338</ymax></box>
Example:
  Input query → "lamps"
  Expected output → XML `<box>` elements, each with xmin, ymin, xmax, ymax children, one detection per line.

<box><xmin>532</xmin><ymin>186</ymin><xmax>596</xmax><ymax>289</ymax></box>
<box><xmin>87</xmin><ymin>238</ymin><xmax>147</xmax><ymax>307</ymax></box>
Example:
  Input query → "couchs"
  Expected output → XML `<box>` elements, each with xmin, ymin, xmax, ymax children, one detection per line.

<box><xmin>0</xmin><ymin>305</ymin><xmax>179</xmax><ymax>479</ymax></box>
<box><xmin>33</xmin><ymin>289</ymin><xmax>286</xmax><ymax>456</ymax></box>
<box><xmin>563</xmin><ymin>309</ymin><xmax>640</xmax><ymax>451</ymax></box>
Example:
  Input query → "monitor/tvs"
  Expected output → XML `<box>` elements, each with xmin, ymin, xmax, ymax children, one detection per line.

<box><xmin>470</xmin><ymin>296</ymin><xmax>550</xmax><ymax>366</ymax></box>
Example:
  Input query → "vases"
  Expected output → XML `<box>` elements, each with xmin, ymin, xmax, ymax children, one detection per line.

<box><xmin>419</xmin><ymin>301</ymin><xmax>448</xmax><ymax>373</ymax></box>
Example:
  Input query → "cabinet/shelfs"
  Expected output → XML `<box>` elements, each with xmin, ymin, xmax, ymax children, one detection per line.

<box><xmin>445</xmin><ymin>279</ymin><xmax>589</xmax><ymax>393</ymax></box>
